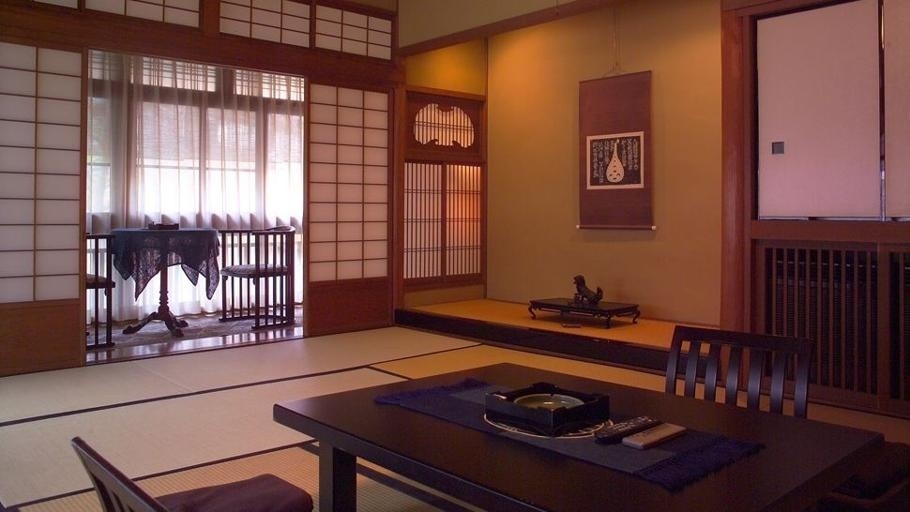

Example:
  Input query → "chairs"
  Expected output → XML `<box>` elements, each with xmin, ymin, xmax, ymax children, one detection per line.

<box><xmin>218</xmin><ymin>225</ymin><xmax>296</xmax><ymax>330</ymax></box>
<box><xmin>71</xmin><ymin>436</ymin><xmax>314</xmax><ymax>512</ymax></box>
<box><xmin>86</xmin><ymin>233</ymin><xmax>116</xmax><ymax>350</ymax></box>
<box><xmin>665</xmin><ymin>325</ymin><xmax>814</xmax><ymax>419</ymax></box>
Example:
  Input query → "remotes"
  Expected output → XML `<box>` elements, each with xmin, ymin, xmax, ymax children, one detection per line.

<box><xmin>622</xmin><ymin>421</ymin><xmax>688</xmax><ymax>451</ymax></box>
<box><xmin>594</xmin><ymin>413</ymin><xmax>660</xmax><ymax>440</ymax></box>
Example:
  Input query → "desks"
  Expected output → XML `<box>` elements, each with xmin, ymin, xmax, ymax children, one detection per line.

<box><xmin>109</xmin><ymin>227</ymin><xmax>220</xmax><ymax>337</ymax></box>
<box><xmin>529</xmin><ymin>297</ymin><xmax>641</xmax><ymax>330</ymax></box>
<box><xmin>273</xmin><ymin>363</ymin><xmax>886</xmax><ymax>512</ymax></box>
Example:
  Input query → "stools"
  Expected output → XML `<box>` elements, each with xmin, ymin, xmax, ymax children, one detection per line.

<box><xmin>817</xmin><ymin>441</ymin><xmax>910</xmax><ymax>511</ymax></box>
